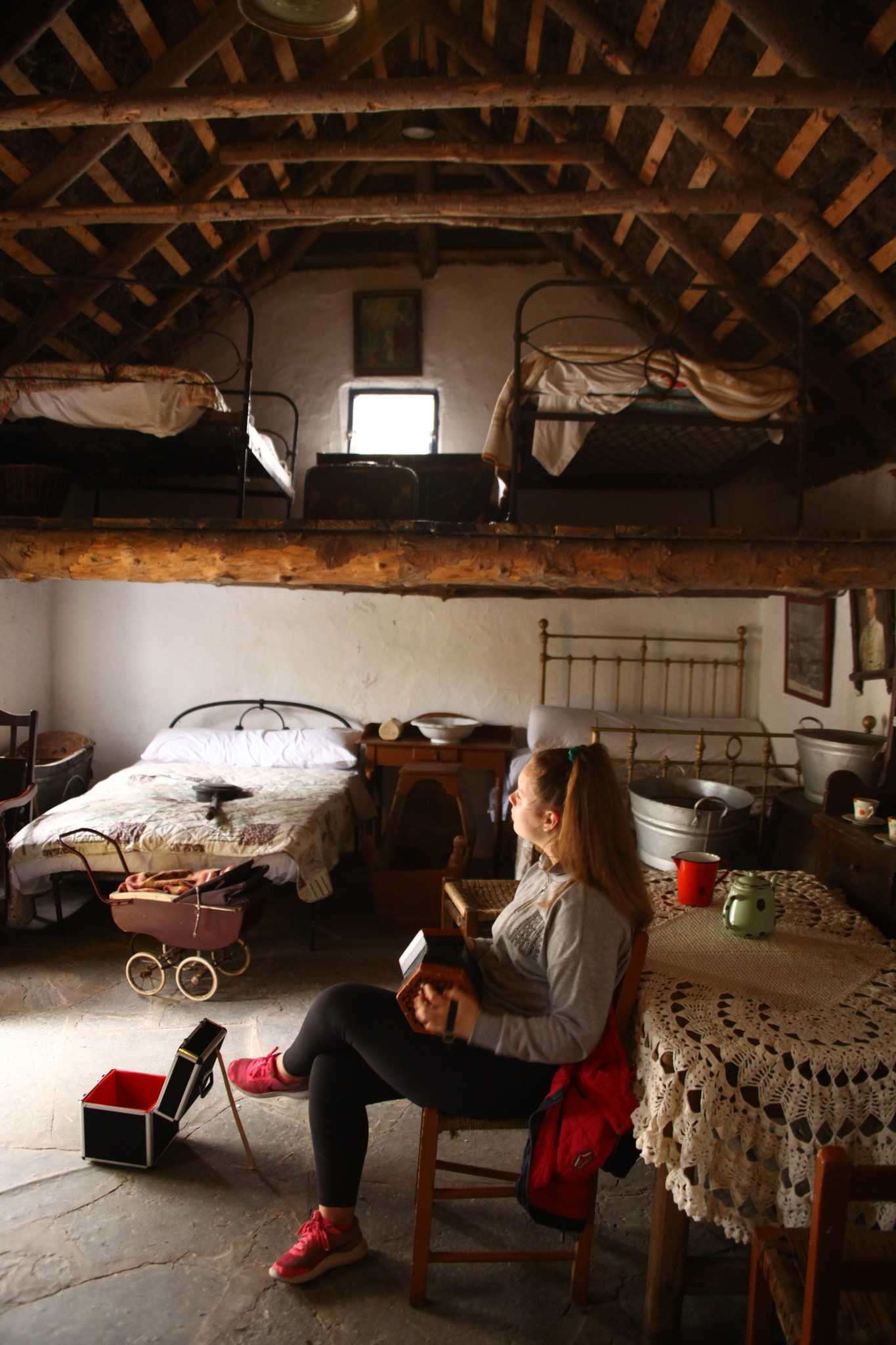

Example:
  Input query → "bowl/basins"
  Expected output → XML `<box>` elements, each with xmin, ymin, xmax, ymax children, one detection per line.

<box><xmin>410</xmin><ymin>717</ymin><xmax>483</xmax><ymax>744</ymax></box>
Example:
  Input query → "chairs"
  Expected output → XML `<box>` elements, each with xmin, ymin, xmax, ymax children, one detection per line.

<box><xmin>745</xmin><ymin>1145</ymin><xmax>896</xmax><ymax>1345</ymax></box>
<box><xmin>0</xmin><ymin>709</ymin><xmax>39</xmax><ymax>918</ymax></box>
<box><xmin>404</xmin><ymin>932</ymin><xmax>651</xmax><ymax>1302</ymax></box>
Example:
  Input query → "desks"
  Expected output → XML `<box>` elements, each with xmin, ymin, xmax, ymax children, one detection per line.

<box><xmin>627</xmin><ymin>871</ymin><xmax>896</xmax><ymax>1345</ymax></box>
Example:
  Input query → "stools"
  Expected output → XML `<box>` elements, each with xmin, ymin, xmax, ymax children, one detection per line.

<box><xmin>382</xmin><ymin>762</ymin><xmax>476</xmax><ymax>867</ymax></box>
<box><xmin>442</xmin><ymin>879</ymin><xmax>520</xmax><ymax>938</ymax></box>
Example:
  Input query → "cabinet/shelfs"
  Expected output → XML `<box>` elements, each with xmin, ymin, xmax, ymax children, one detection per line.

<box><xmin>354</xmin><ymin>723</ymin><xmax>515</xmax><ymax>873</ymax></box>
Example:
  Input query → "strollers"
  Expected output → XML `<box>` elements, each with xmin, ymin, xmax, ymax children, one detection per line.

<box><xmin>57</xmin><ymin>828</ymin><xmax>252</xmax><ymax>1001</ymax></box>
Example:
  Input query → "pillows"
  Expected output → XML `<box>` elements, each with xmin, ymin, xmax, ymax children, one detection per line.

<box><xmin>527</xmin><ymin>705</ymin><xmax>767</xmax><ymax>763</ymax></box>
<box><xmin>141</xmin><ymin>729</ymin><xmax>358</xmax><ymax>770</ymax></box>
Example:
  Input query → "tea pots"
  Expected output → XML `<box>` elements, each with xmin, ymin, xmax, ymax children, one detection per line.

<box><xmin>722</xmin><ymin>870</ymin><xmax>779</xmax><ymax>939</ymax></box>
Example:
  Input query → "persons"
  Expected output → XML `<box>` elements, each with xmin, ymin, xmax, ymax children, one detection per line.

<box><xmin>229</xmin><ymin>742</ymin><xmax>655</xmax><ymax>1292</ymax></box>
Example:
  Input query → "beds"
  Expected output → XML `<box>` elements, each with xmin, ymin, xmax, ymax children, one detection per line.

<box><xmin>0</xmin><ymin>276</ymin><xmax>298</xmax><ymax>522</ymax></box>
<box><xmin>7</xmin><ymin>699</ymin><xmax>378</xmax><ymax>951</ymax></box>
<box><xmin>512</xmin><ymin>617</ymin><xmax>876</xmax><ymax>850</ymax></box>
<box><xmin>480</xmin><ymin>278</ymin><xmax>809</xmax><ymax>531</ymax></box>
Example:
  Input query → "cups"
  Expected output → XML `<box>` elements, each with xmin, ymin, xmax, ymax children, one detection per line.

<box><xmin>853</xmin><ymin>798</ymin><xmax>879</xmax><ymax>822</ymax></box>
<box><xmin>887</xmin><ymin>816</ymin><xmax>896</xmax><ymax>842</ymax></box>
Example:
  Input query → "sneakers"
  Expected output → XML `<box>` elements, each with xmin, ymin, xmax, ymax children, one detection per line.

<box><xmin>229</xmin><ymin>1045</ymin><xmax>310</xmax><ymax>1100</ymax></box>
<box><xmin>269</xmin><ymin>1209</ymin><xmax>370</xmax><ymax>1284</ymax></box>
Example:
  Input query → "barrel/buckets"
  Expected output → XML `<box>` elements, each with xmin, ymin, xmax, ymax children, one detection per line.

<box><xmin>625</xmin><ymin>764</ymin><xmax>755</xmax><ymax>870</ymax></box>
<box><xmin>792</xmin><ymin>716</ymin><xmax>887</xmax><ymax>804</ymax></box>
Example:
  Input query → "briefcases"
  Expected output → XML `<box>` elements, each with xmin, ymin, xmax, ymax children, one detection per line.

<box><xmin>81</xmin><ymin>1018</ymin><xmax>228</xmax><ymax>1172</ymax></box>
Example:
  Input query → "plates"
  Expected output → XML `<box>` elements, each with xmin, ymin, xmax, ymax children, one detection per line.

<box><xmin>874</xmin><ymin>833</ymin><xmax>896</xmax><ymax>847</ymax></box>
<box><xmin>841</xmin><ymin>813</ymin><xmax>886</xmax><ymax>826</ymax></box>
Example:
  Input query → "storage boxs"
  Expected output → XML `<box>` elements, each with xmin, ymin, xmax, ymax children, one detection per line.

<box><xmin>81</xmin><ymin>1016</ymin><xmax>226</xmax><ymax>1171</ymax></box>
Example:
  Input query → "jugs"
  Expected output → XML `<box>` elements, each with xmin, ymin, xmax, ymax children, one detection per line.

<box><xmin>670</xmin><ymin>851</ymin><xmax>730</xmax><ymax>908</ymax></box>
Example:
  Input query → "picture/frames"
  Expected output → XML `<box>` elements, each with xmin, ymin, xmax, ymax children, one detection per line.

<box><xmin>782</xmin><ymin>595</ymin><xmax>835</xmax><ymax>707</ymax></box>
<box><xmin>352</xmin><ymin>290</ymin><xmax>424</xmax><ymax>376</ymax></box>
